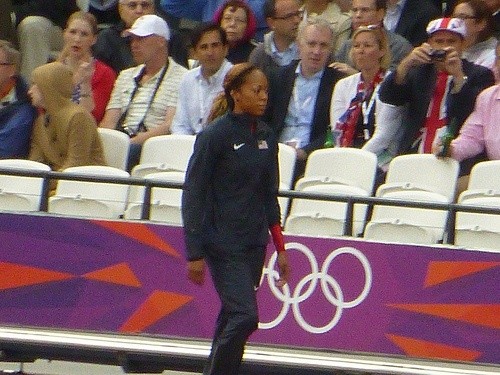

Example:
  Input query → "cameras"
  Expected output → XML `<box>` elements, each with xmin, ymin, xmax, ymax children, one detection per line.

<box><xmin>429</xmin><ymin>49</ymin><xmax>448</xmax><ymax>61</ymax></box>
<box><xmin>120</xmin><ymin>125</ymin><xmax>134</xmax><ymax>136</ymax></box>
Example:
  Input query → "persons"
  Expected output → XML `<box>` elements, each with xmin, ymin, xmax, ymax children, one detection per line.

<box><xmin>0</xmin><ymin>0</ymin><xmax>500</xmax><ymax>244</ymax></box>
<box><xmin>181</xmin><ymin>63</ymin><xmax>287</xmax><ymax>375</ymax></box>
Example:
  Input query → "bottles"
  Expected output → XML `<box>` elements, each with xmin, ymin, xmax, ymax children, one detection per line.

<box><xmin>439</xmin><ymin>117</ymin><xmax>456</xmax><ymax>157</ymax></box>
<box><xmin>324</xmin><ymin>125</ymin><xmax>334</xmax><ymax>148</ymax></box>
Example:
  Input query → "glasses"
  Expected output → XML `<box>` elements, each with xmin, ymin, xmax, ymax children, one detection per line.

<box><xmin>357</xmin><ymin>25</ymin><xmax>385</xmax><ymax>39</ymax></box>
<box><xmin>457</xmin><ymin>14</ymin><xmax>477</xmax><ymax>20</ymax></box>
<box><xmin>273</xmin><ymin>11</ymin><xmax>304</xmax><ymax>22</ymax></box>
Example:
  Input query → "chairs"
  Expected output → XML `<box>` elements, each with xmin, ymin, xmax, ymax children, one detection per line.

<box><xmin>0</xmin><ymin>125</ymin><xmax>500</xmax><ymax>253</ymax></box>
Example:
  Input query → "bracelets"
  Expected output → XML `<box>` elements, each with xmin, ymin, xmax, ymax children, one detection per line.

<box><xmin>456</xmin><ymin>75</ymin><xmax>468</xmax><ymax>82</ymax></box>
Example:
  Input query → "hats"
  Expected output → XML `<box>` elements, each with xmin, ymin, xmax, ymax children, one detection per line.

<box><xmin>426</xmin><ymin>17</ymin><xmax>467</xmax><ymax>39</ymax></box>
<box><xmin>120</xmin><ymin>15</ymin><xmax>171</xmax><ymax>41</ymax></box>
